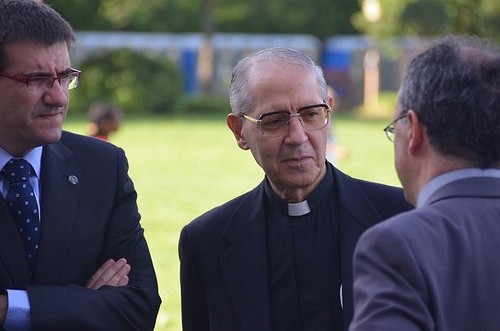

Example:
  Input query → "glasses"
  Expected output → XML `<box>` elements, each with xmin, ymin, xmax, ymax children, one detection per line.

<box><xmin>0</xmin><ymin>66</ymin><xmax>83</xmax><ymax>96</ymax></box>
<box><xmin>234</xmin><ymin>100</ymin><xmax>332</xmax><ymax>139</ymax></box>
<box><xmin>384</xmin><ymin>114</ymin><xmax>408</xmax><ymax>142</ymax></box>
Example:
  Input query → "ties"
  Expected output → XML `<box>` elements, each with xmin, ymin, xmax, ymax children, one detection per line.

<box><xmin>0</xmin><ymin>158</ymin><xmax>40</xmax><ymax>268</ymax></box>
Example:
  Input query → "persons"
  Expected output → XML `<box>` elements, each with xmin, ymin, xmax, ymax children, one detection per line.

<box><xmin>178</xmin><ymin>47</ymin><xmax>416</xmax><ymax>331</ymax></box>
<box><xmin>349</xmin><ymin>33</ymin><xmax>500</xmax><ymax>331</ymax></box>
<box><xmin>0</xmin><ymin>0</ymin><xmax>161</xmax><ymax>331</ymax></box>
